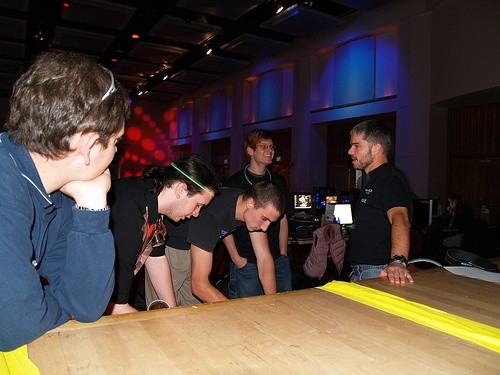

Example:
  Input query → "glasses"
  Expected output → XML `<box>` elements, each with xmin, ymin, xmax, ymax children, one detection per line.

<box><xmin>256</xmin><ymin>144</ymin><xmax>276</xmax><ymax>150</ymax></box>
<box><xmin>80</xmin><ymin>62</ymin><xmax>120</xmax><ymax>125</ymax></box>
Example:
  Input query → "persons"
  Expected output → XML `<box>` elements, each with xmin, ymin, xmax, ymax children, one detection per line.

<box><xmin>106</xmin><ymin>155</ymin><xmax>220</xmax><ymax>314</ymax></box>
<box><xmin>447</xmin><ymin>194</ymin><xmax>479</xmax><ymax>232</ymax></box>
<box><xmin>344</xmin><ymin>119</ymin><xmax>414</xmax><ymax>283</ymax></box>
<box><xmin>222</xmin><ymin>128</ymin><xmax>292</xmax><ymax>299</ymax></box>
<box><xmin>0</xmin><ymin>43</ymin><xmax>129</xmax><ymax>352</ymax></box>
<box><xmin>145</xmin><ymin>179</ymin><xmax>287</xmax><ymax>310</ymax></box>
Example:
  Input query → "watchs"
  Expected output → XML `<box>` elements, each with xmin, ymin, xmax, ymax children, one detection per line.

<box><xmin>389</xmin><ymin>254</ymin><xmax>409</xmax><ymax>269</ymax></box>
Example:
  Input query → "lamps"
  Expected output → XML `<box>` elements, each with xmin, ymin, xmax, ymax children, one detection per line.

<box><xmin>271</xmin><ymin>0</ymin><xmax>283</xmax><ymax>15</ymax></box>
<box><xmin>204</xmin><ymin>43</ymin><xmax>212</xmax><ymax>56</ymax></box>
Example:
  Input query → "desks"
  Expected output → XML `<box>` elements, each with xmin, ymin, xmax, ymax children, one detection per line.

<box><xmin>421</xmin><ymin>227</ymin><xmax>460</xmax><ymax>233</ymax></box>
<box><xmin>285</xmin><ymin>235</ymin><xmax>349</xmax><ymax>266</ymax></box>
<box><xmin>0</xmin><ymin>265</ymin><xmax>500</xmax><ymax>375</ymax></box>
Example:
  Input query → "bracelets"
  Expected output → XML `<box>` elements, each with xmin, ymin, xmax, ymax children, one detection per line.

<box><xmin>74</xmin><ymin>204</ymin><xmax>110</xmax><ymax>211</ymax></box>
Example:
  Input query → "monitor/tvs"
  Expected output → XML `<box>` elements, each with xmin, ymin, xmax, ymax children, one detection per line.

<box><xmin>293</xmin><ymin>193</ymin><xmax>312</xmax><ymax>210</ymax></box>
<box><xmin>327</xmin><ymin>202</ymin><xmax>354</xmax><ymax>230</ymax></box>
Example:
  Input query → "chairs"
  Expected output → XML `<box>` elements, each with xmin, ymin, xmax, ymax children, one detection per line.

<box><xmin>289</xmin><ymin>224</ymin><xmax>349</xmax><ymax>290</ymax></box>
<box><xmin>416</xmin><ymin>222</ymin><xmax>443</xmax><ymax>268</ymax></box>
<box><xmin>461</xmin><ymin>218</ymin><xmax>491</xmax><ymax>259</ymax></box>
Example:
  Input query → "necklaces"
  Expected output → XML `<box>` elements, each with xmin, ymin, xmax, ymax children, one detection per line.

<box><xmin>244</xmin><ymin>164</ymin><xmax>271</xmax><ymax>185</ymax></box>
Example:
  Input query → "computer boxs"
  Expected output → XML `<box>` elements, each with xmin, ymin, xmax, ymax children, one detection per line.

<box><xmin>411</xmin><ymin>198</ymin><xmax>438</xmax><ymax>229</ymax></box>
